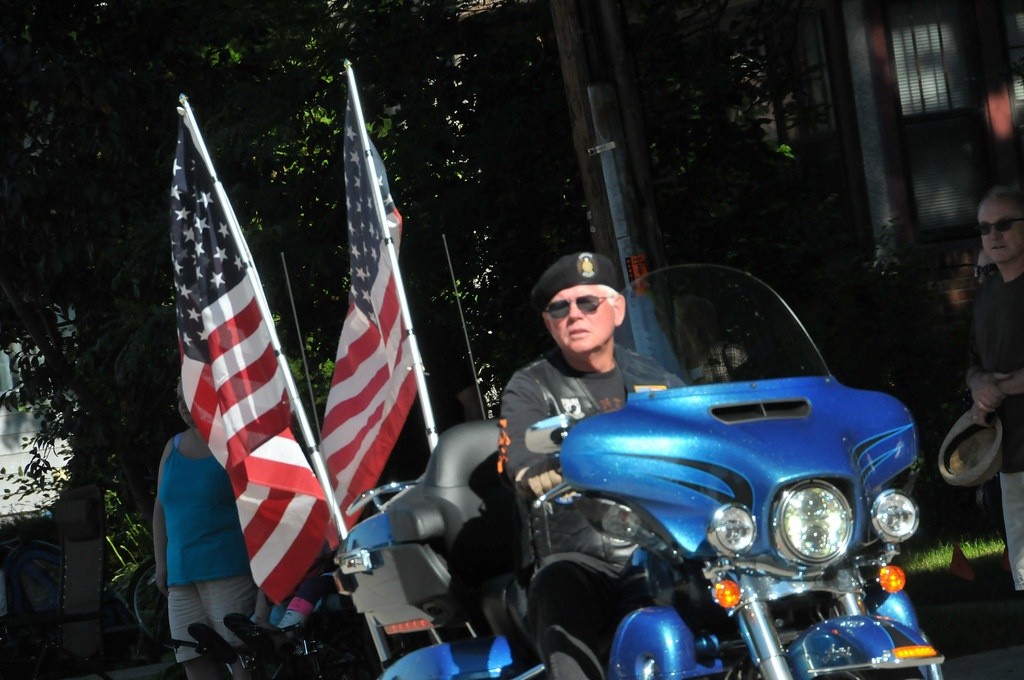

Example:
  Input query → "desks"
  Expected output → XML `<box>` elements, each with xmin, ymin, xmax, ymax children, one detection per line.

<box><xmin>0</xmin><ymin>611</ymin><xmax>113</xmax><ymax>680</ymax></box>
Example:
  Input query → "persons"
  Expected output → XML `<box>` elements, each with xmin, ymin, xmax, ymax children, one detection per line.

<box><xmin>964</xmin><ymin>184</ymin><xmax>1024</xmax><ymax>592</ymax></box>
<box><xmin>501</xmin><ymin>252</ymin><xmax>689</xmax><ymax>583</ymax></box>
<box><xmin>152</xmin><ymin>373</ymin><xmax>344</xmax><ymax>680</ymax></box>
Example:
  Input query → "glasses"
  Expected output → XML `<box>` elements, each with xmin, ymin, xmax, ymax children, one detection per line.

<box><xmin>978</xmin><ymin>217</ymin><xmax>1024</xmax><ymax>235</ymax></box>
<box><xmin>538</xmin><ymin>296</ymin><xmax>619</xmax><ymax>319</ymax></box>
<box><xmin>972</xmin><ymin>263</ymin><xmax>998</xmax><ymax>278</ymax></box>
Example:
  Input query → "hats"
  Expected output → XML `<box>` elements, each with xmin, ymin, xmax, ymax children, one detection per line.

<box><xmin>938</xmin><ymin>408</ymin><xmax>1001</xmax><ymax>486</ymax></box>
<box><xmin>529</xmin><ymin>252</ymin><xmax>619</xmax><ymax>316</ymax></box>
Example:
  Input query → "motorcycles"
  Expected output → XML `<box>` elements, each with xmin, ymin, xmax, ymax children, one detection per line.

<box><xmin>332</xmin><ymin>262</ymin><xmax>948</xmax><ymax>680</ymax></box>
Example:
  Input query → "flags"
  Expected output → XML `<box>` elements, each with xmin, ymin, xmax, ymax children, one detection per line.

<box><xmin>320</xmin><ymin>79</ymin><xmax>425</xmax><ymax>551</ymax></box>
<box><xmin>168</xmin><ymin>102</ymin><xmax>329</xmax><ymax>606</ymax></box>
